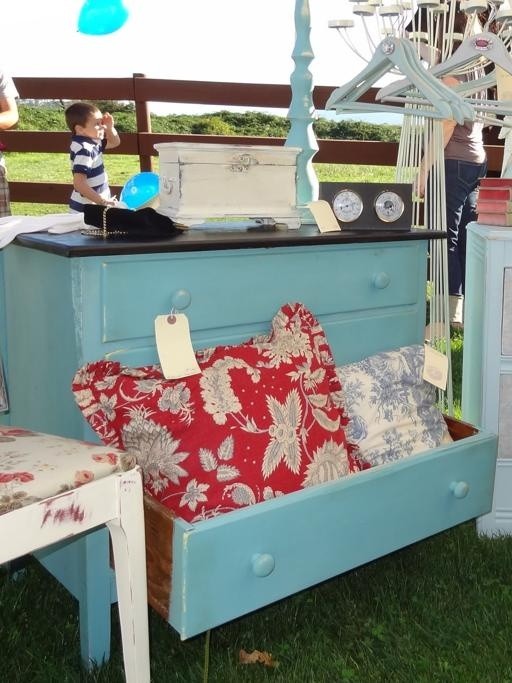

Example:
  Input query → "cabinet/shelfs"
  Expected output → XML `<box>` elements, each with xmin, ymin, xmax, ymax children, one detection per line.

<box><xmin>0</xmin><ymin>201</ymin><xmax>502</xmax><ymax>675</ymax></box>
<box><xmin>462</xmin><ymin>218</ymin><xmax>512</xmax><ymax>540</ymax></box>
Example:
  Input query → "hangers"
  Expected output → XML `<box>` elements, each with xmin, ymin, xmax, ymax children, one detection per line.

<box><xmin>325</xmin><ymin>0</ymin><xmax>512</xmax><ymax>127</ymax></box>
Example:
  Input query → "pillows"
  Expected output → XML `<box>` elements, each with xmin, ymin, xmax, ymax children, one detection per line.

<box><xmin>333</xmin><ymin>344</ymin><xmax>459</xmax><ymax>469</ymax></box>
<box><xmin>72</xmin><ymin>303</ymin><xmax>367</xmax><ymax>523</ymax></box>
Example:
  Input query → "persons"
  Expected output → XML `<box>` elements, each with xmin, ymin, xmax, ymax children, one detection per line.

<box><xmin>64</xmin><ymin>102</ymin><xmax>121</xmax><ymax>214</ymax></box>
<box><xmin>413</xmin><ymin>13</ymin><xmax>488</xmax><ymax>342</ymax></box>
<box><xmin>0</xmin><ymin>67</ymin><xmax>21</xmax><ymax>216</ymax></box>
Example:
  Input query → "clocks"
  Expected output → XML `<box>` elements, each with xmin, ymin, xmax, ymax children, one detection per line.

<box><xmin>318</xmin><ymin>181</ymin><xmax>411</xmax><ymax>231</ymax></box>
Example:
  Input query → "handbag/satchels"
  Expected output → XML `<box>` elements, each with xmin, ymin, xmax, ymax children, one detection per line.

<box><xmin>81</xmin><ymin>203</ymin><xmax>189</xmax><ymax>238</ymax></box>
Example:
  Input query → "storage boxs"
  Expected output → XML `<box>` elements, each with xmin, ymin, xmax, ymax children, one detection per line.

<box><xmin>154</xmin><ymin>141</ymin><xmax>303</xmax><ymax>231</ymax></box>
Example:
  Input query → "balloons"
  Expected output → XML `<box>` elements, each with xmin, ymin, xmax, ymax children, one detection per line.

<box><xmin>118</xmin><ymin>171</ymin><xmax>159</xmax><ymax>211</ymax></box>
<box><xmin>76</xmin><ymin>0</ymin><xmax>131</xmax><ymax>36</ymax></box>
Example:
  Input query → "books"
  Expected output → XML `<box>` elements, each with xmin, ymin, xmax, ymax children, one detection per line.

<box><xmin>476</xmin><ymin>177</ymin><xmax>511</xmax><ymax>228</ymax></box>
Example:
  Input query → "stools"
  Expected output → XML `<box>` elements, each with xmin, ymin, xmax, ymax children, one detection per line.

<box><xmin>0</xmin><ymin>426</ymin><xmax>155</xmax><ymax>683</ymax></box>
